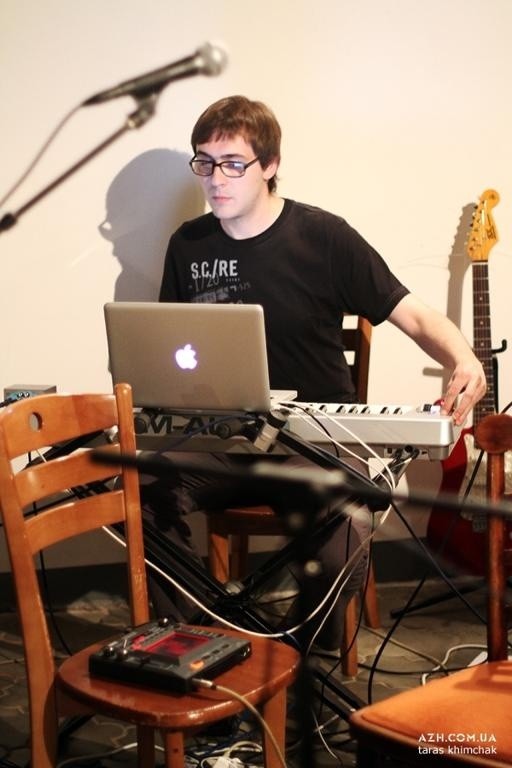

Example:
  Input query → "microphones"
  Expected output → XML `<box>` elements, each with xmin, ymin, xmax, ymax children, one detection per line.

<box><xmin>83</xmin><ymin>42</ymin><xmax>225</xmax><ymax>109</ymax></box>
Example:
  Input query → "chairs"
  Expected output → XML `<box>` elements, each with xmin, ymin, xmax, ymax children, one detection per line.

<box><xmin>209</xmin><ymin>313</ymin><xmax>373</xmax><ymax>678</ymax></box>
<box><xmin>0</xmin><ymin>383</ymin><xmax>302</xmax><ymax>768</ymax></box>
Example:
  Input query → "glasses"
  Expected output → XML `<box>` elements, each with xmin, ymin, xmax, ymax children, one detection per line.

<box><xmin>188</xmin><ymin>152</ymin><xmax>262</xmax><ymax>179</ymax></box>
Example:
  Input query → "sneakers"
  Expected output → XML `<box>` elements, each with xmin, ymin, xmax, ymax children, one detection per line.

<box><xmin>297</xmin><ymin>657</ymin><xmax>342</xmax><ymax>741</ymax></box>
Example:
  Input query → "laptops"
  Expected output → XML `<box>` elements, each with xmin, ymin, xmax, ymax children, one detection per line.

<box><xmin>103</xmin><ymin>301</ymin><xmax>298</xmax><ymax>414</ymax></box>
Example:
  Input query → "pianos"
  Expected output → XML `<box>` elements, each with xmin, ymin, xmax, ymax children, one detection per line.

<box><xmin>2</xmin><ymin>383</ymin><xmax>464</xmax><ymax>462</ymax></box>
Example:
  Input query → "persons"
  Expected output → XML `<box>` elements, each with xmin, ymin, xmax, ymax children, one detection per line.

<box><xmin>113</xmin><ymin>94</ymin><xmax>486</xmax><ymax>742</ymax></box>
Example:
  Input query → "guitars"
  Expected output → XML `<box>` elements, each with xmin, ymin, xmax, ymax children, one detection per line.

<box><xmin>426</xmin><ymin>188</ymin><xmax>512</xmax><ymax>573</ymax></box>
